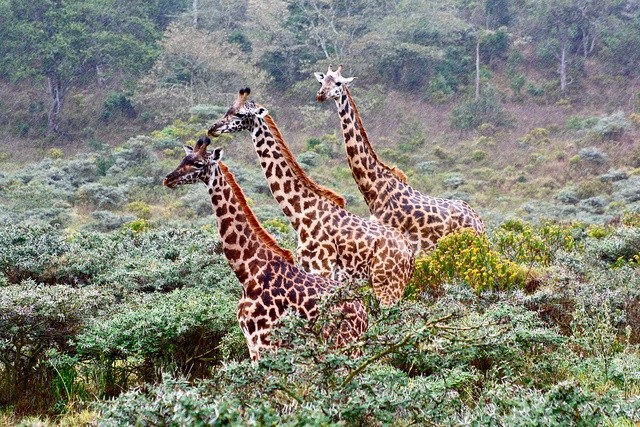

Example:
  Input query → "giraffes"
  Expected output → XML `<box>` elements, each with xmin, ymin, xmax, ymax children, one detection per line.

<box><xmin>162</xmin><ymin>134</ymin><xmax>369</xmax><ymax>370</ymax></box>
<box><xmin>314</xmin><ymin>62</ymin><xmax>489</xmax><ymax>286</ymax></box>
<box><xmin>208</xmin><ymin>85</ymin><xmax>415</xmax><ymax>313</ymax></box>
